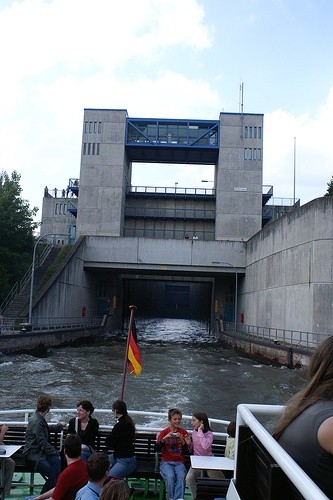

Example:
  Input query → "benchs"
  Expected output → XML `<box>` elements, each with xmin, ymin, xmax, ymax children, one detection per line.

<box><xmin>1</xmin><ymin>426</ymin><xmax>226</xmax><ymax>499</ymax></box>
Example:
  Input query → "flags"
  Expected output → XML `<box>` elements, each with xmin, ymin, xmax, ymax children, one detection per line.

<box><xmin>127</xmin><ymin>313</ymin><xmax>143</xmax><ymax>377</ymax></box>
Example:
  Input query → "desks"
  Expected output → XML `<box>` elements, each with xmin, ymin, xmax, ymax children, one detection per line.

<box><xmin>0</xmin><ymin>444</ymin><xmax>22</xmax><ymax>500</ymax></box>
<box><xmin>190</xmin><ymin>456</ymin><xmax>234</xmax><ymax>499</ymax></box>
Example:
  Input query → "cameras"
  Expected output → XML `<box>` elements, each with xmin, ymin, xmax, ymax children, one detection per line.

<box><xmin>172</xmin><ymin>433</ymin><xmax>178</xmax><ymax>438</ymax></box>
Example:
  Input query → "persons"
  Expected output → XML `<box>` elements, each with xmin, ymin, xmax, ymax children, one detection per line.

<box><xmin>66</xmin><ymin>400</ymin><xmax>99</xmax><ymax>462</ymax></box>
<box><xmin>183</xmin><ymin>412</ymin><xmax>226</xmax><ymax>500</ymax></box>
<box><xmin>154</xmin><ymin>408</ymin><xmax>191</xmax><ymax>500</ymax></box>
<box><xmin>225</xmin><ymin>420</ymin><xmax>236</xmax><ymax>478</ymax></box>
<box><xmin>74</xmin><ymin>454</ymin><xmax>110</xmax><ymax>500</ymax></box>
<box><xmin>0</xmin><ymin>424</ymin><xmax>16</xmax><ymax>500</ymax></box>
<box><xmin>32</xmin><ymin>434</ymin><xmax>89</xmax><ymax>500</ymax></box>
<box><xmin>22</xmin><ymin>393</ymin><xmax>67</xmax><ymax>494</ymax></box>
<box><xmin>44</xmin><ymin>180</ymin><xmax>78</xmax><ymax>198</ymax></box>
<box><xmin>105</xmin><ymin>400</ymin><xmax>137</xmax><ymax>479</ymax></box>
<box><xmin>270</xmin><ymin>336</ymin><xmax>333</xmax><ymax>500</ymax></box>
<box><xmin>100</xmin><ymin>480</ymin><xmax>130</xmax><ymax>500</ymax></box>
